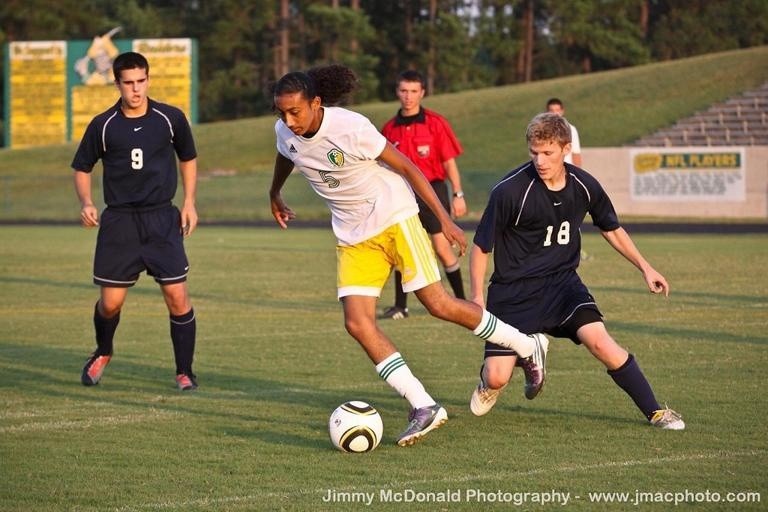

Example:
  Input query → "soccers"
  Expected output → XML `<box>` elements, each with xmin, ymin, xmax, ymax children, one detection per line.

<box><xmin>329</xmin><ymin>402</ymin><xmax>383</xmax><ymax>454</ymax></box>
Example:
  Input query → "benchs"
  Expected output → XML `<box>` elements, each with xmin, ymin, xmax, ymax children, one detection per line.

<box><xmin>632</xmin><ymin>80</ymin><xmax>768</xmax><ymax>145</ymax></box>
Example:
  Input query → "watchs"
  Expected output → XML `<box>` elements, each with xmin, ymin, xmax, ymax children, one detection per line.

<box><xmin>452</xmin><ymin>190</ymin><xmax>465</xmax><ymax>198</ymax></box>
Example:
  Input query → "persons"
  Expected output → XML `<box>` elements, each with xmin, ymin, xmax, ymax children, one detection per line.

<box><xmin>468</xmin><ymin>112</ymin><xmax>687</xmax><ymax>433</ymax></box>
<box><xmin>376</xmin><ymin>68</ymin><xmax>468</xmax><ymax>321</ymax></box>
<box><xmin>70</xmin><ymin>51</ymin><xmax>201</xmax><ymax>392</ymax></box>
<box><xmin>264</xmin><ymin>63</ymin><xmax>549</xmax><ymax>448</ymax></box>
<box><xmin>545</xmin><ymin>96</ymin><xmax>581</xmax><ymax>170</ymax></box>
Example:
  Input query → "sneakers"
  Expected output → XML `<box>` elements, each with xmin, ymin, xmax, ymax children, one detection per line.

<box><xmin>82</xmin><ymin>348</ymin><xmax>112</xmax><ymax>383</ymax></box>
<box><xmin>396</xmin><ymin>401</ymin><xmax>447</xmax><ymax>446</ymax></box>
<box><xmin>647</xmin><ymin>402</ymin><xmax>685</xmax><ymax>430</ymax></box>
<box><xmin>472</xmin><ymin>381</ymin><xmax>509</xmax><ymax>417</ymax></box>
<box><xmin>380</xmin><ymin>306</ymin><xmax>408</xmax><ymax>321</ymax></box>
<box><xmin>173</xmin><ymin>372</ymin><xmax>195</xmax><ymax>390</ymax></box>
<box><xmin>524</xmin><ymin>333</ymin><xmax>549</xmax><ymax>399</ymax></box>
